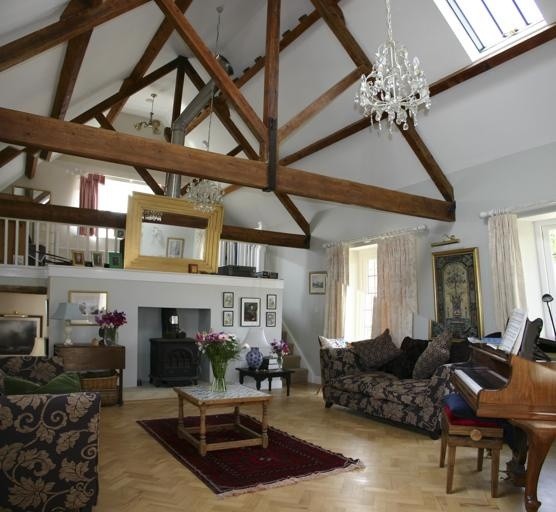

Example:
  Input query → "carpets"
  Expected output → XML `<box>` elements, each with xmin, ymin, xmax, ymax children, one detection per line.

<box><xmin>135</xmin><ymin>413</ymin><xmax>365</xmax><ymax>499</ymax></box>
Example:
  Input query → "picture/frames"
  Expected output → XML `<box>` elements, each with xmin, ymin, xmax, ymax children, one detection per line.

<box><xmin>71</xmin><ymin>250</ymin><xmax>123</xmax><ymax>269</ymax></box>
<box><xmin>223</xmin><ymin>292</ymin><xmax>277</xmax><ymax>327</ymax></box>
<box><xmin>0</xmin><ymin>315</ymin><xmax>43</xmax><ymax>355</ymax></box>
<box><xmin>431</xmin><ymin>247</ymin><xmax>484</xmax><ymax>343</ymax></box>
<box><xmin>166</xmin><ymin>237</ymin><xmax>185</xmax><ymax>257</ymax></box>
<box><xmin>309</xmin><ymin>271</ymin><xmax>327</xmax><ymax>295</ymax></box>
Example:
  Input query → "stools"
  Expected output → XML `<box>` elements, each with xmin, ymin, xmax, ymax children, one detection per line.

<box><xmin>437</xmin><ymin>395</ymin><xmax>505</xmax><ymax>498</ymax></box>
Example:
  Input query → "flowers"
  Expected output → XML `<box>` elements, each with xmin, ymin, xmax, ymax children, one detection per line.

<box><xmin>271</xmin><ymin>339</ymin><xmax>290</xmax><ymax>367</ymax></box>
<box><xmin>195</xmin><ymin>328</ymin><xmax>242</xmax><ymax>392</ymax></box>
<box><xmin>95</xmin><ymin>310</ymin><xmax>127</xmax><ymax>344</ymax></box>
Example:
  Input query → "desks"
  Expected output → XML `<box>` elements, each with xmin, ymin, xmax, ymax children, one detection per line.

<box><xmin>54</xmin><ymin>343</ymin><xmax>125</xmax><ymax>407</ymax></box>
<box><xmin>173</xmin><ymin>386</ymin><xmax>274</xmax><ymax>457</ymax></box>
<box><xmin>236</xmin><ymin>368</ymin><xmax>295</xmax><ymax>396</ymax></box>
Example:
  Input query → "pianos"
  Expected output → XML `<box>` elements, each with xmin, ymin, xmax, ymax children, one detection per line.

<box><xmin>449</xmin><ymin>331</ymin><xmax>556</xmax><ymax>508</ymax></box>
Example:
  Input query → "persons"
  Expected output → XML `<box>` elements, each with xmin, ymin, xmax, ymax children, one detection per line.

<box><xmin>247</xmin><ymin>303</ymin><xmax>256</xmax><ymax>320</ymax></box>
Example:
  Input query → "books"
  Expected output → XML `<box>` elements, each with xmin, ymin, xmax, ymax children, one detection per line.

<box><xmin>499</xmin><ymin>310</ymin><xmax>527</xmax><ymax>355</ymax></box>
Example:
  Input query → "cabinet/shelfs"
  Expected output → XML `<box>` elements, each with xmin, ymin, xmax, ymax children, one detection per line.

<box><xmin>150</xmin><ymin>337</ymin><xmax>197</xmax><ymax>386</ymax></box>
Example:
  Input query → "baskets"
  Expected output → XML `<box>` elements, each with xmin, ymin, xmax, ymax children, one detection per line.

<box><xmin>84</xmin><ymin>389</ymin><xmax>119</xmax><ymax>407</ymax></box>
<box><xmin>79</xmin><ymin>369</ymin><xmax>120</xmax><ymax>390</ymax></box>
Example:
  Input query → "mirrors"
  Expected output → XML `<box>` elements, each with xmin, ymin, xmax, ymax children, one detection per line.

<box><xmin>68</xmin><ymin>290</ymin><xmax>109</xmax><ymax>326</ymax></box>
<box><xmin>12</xmin><ymin>185</ymin><xmax>52</xmax><ymax>205</ymax></box>
<box><xmin>123</xmin><ymin>194</ymin><xmax>224</xmax><ymax>274</ymax></box>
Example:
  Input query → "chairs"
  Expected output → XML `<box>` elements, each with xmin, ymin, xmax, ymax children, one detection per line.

<box><xmin>0</xmin><ymin>352</ymin><xmax>101</xmax><ymax>512</ymax></box>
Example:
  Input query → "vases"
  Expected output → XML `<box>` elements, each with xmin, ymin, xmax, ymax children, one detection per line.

<box><xmin>103</xmin><ymin>328</ymin><xmax>117</xmax><ymax>347</ymax></box>
<box><xmin>211</xmin><ymin>361</ymin><xmax>228</xmax><ymax>393</ymax></box>
<box><xmin>277</xmin><ymin>357</ymin><xmax>284</xmax><ymax>369</ymax></box>
<box><xmin>246</xmin><ymin>347</ymin><xmax>264</xmax><ymax>369</ymax></box>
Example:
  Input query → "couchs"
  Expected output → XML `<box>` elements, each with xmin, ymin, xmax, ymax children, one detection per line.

<box><xmin>317</xmin><ymin>328</ymin><xmax>502</xmax><ymax>440</ymax></box>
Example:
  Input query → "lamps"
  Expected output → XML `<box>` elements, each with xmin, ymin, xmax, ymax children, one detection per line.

<box><xmin>542</xmin><ymin>294</ymin><xmax>556</xmax><ymax>337</ymax></box>
<box><xmin>50</xmin><ymin>302</ymin><xmax>87</xmax><ymax>347</ymax></box>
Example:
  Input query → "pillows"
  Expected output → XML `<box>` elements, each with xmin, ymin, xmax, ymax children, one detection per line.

<box><xmin>4</xmin><ymin>371</ymin><xmax>81</xmax><ymax>392</ymax></box>
<box><xmin>347</xmin><ymin>329</ymin><xmax>454</xmax><ymax>381</ymax></box>
